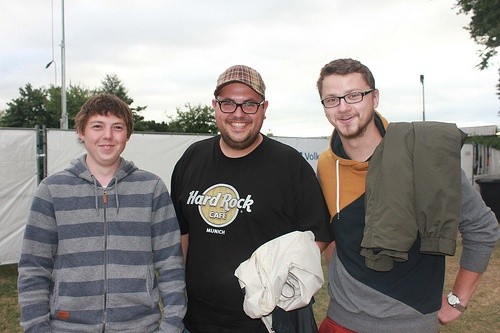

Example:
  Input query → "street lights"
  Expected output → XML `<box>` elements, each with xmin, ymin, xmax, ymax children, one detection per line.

<box><xmin>420</xmin><ymin>75</ymin><xmax>425</xmax><ymax>122</ymax></box>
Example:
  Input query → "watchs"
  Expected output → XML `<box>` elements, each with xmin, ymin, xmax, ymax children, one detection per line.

<box><xmin>446</xmin><ymin>292</ymin><xmax>466</xmax><ymax>313</ymax></box>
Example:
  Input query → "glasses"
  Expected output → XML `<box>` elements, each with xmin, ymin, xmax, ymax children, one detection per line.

<box><xmin>321</xmin><ymin>89</ymin><xmax>373</xmax><ymax>108</ymax></box>
<box><xmin>217</xmin><ymin>99</ymin><xmax>264</xmax><ymax>114</ymax></box>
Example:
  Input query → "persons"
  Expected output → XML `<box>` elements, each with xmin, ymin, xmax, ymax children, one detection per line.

<box><xmin>16</xmin><ymin>93</ymin><xmax>188</xmax><ymax>333</ymax></box>
<box><xmin>317</xmin><ymin>58</ymin><xmax>500</xmax><ymax>333</ymax></box>
<box><xmin>170</xmin><ymin>65</ymin><xmax>331</xmax><ymax>333</ymax></box>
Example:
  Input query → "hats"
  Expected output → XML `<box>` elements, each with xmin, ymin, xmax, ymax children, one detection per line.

<box><xmin>214</xmin><ymin>65</ymin><xmax>265</xmax><ymax>102</ymax></box>
